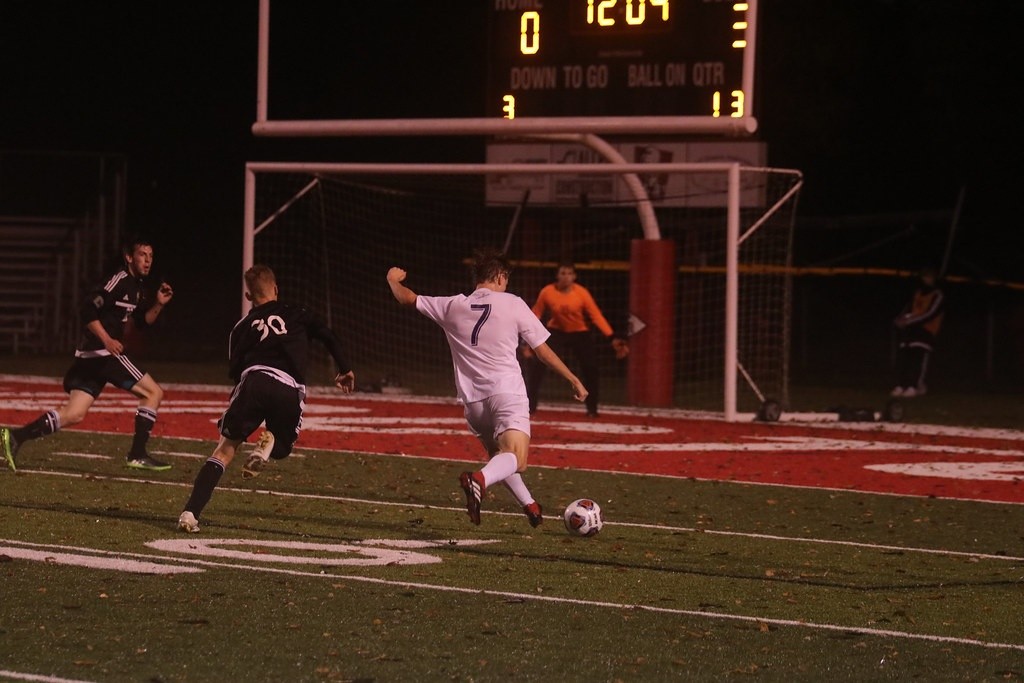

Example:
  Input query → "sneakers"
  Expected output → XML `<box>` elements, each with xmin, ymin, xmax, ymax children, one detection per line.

<box><xmin>522</xmin><ymin>499</ymin><xmax>543</xmax><ymax>529</ymax></box>
<box><xmin>240</xmin><ymin>430</ymin><xmax>275</xmax><ymax>480</ymax></box>
<box><xmin>125</xmin><ymin>452</ymin><xmax>172</xmax><ymax>470</ymax></box>
<box><xmin>176</xmin><ymin>510</ymin><xmax>201</xmax><ymax>533</ymax></box>
<box><xmin>458</xmin><ymin>469</ymin><xmax>486</xmax><ymax>525</ymax></box>
<box><xmin>0</xmin><ymin>428</ymin><xmax>18</xmax><ymax>473</ymax></box>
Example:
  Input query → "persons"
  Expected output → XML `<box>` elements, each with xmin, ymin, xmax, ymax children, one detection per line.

<box><xmin>0</xmin><ymin>239</ymin><xmax>173</xmax><ymax>473</ymax></box>
<box><xmin>521</xmin><ymin>257</ymin><xmax>628</xmax><ymax>418</ymax></box>
<box><xmin>387</xmin><ymin>243</ymin><xmax>590</xmax><ymax>527</ymax></box>
<box><xmin>174</xmin><ymin>270</ymin><xmax>355</xmax><ymax>531</ymax></box>
<box><xmin>891</xmin><ymin>265</ymin><xmax>947</xmax><ymax>397</ymax></box>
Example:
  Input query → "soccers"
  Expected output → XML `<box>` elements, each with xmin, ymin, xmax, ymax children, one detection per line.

<box><xmin>564</xmin><ymin>498</ymin><xmax>604</xmax><ymax>537</ymax></box>
